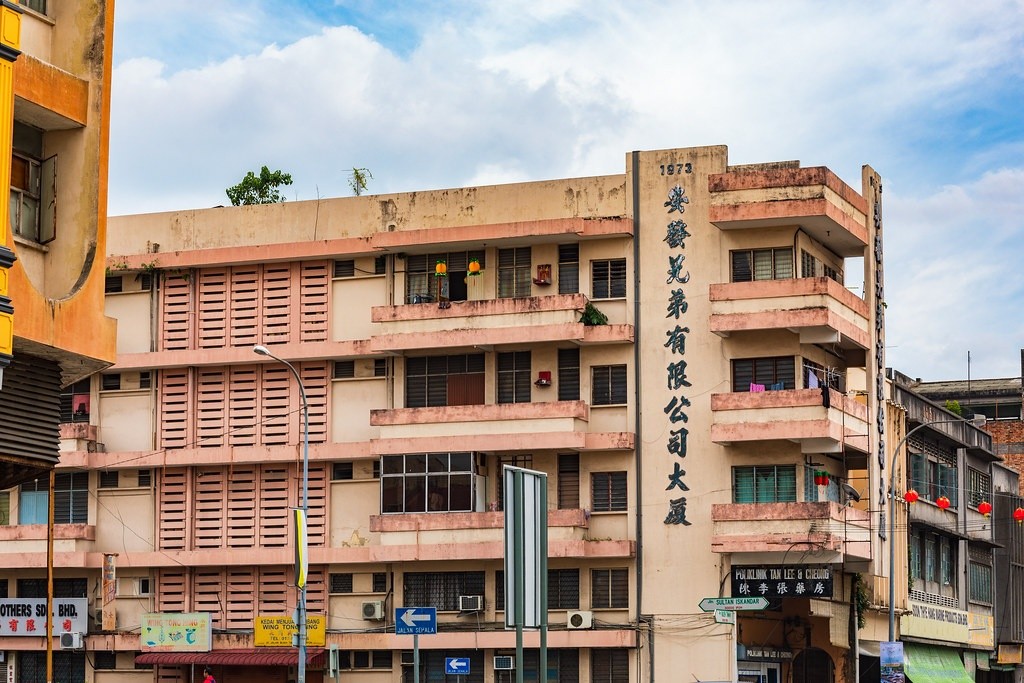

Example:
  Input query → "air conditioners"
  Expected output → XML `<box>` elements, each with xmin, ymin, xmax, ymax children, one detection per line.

<box><xmin>494</xmin><ymin>656</ymin><xmax>516</xmax><ymax>669</ymax></box>
<box><xmin>459</xmin><ymin>596</ymin><xmax>483</xmax><ymax>611</ymax></box>
<box><xmin>362</xmin><ymin>601</ymin><xmax>385</xmax><ymax>619</ymax></box>
<box><xmin>567</xmin><ymin>611</ymin><xmax>593</xmax><ymax>628</ymax></box>
